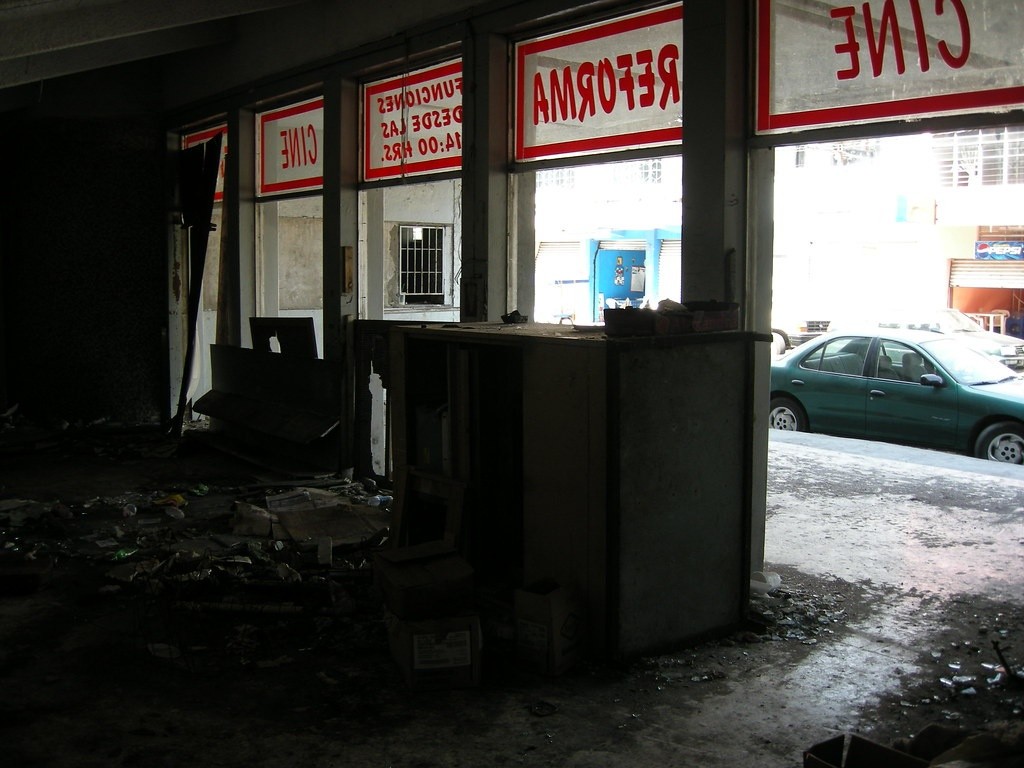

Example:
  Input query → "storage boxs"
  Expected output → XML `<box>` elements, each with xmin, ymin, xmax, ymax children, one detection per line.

<box><xmin>383</xmin><ymin>612</ymin><xmax>486</xmax><ymax>691</ymax></box>
<box><xmin>376</xmin><ymin>540</ymin><xmax>478</xmax><ymax>619</ymax></box>
<box><xmin>665</xmin><ymin>309</ymin><xmax>738</xmax><ymax>334</ymax></box>
<box><xmin>513</xmin><ymin>584</ymin><xmax>583</xmax><ymax>678</ymax></box>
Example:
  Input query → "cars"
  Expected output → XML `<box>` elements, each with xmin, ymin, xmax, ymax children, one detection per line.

<box><xmin>819</xmin><ymin>304</ymin><xmax>1024</xmax><ymax>374</ymax></box>
<box><xmin>768</xmin><ymin>332</ymin><xmax>1024</xmax><ymax>465</ymax></box>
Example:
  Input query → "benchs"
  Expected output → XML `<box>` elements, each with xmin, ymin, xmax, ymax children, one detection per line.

<box><xmin>805</xmin><ymin>353</ymin><xmax>864</xmax><ymax>375</ymax></box>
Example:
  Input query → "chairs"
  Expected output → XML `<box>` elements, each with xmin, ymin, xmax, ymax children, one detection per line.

<box><xmin>902</xmin><ymin>352</ymin><xmax>928</xmax><ymax>382</ymax></box>
<box><xmin>879</xmin><ymin>355</ymin><xmax>900</xmax><ymax>381</ymax></box>
